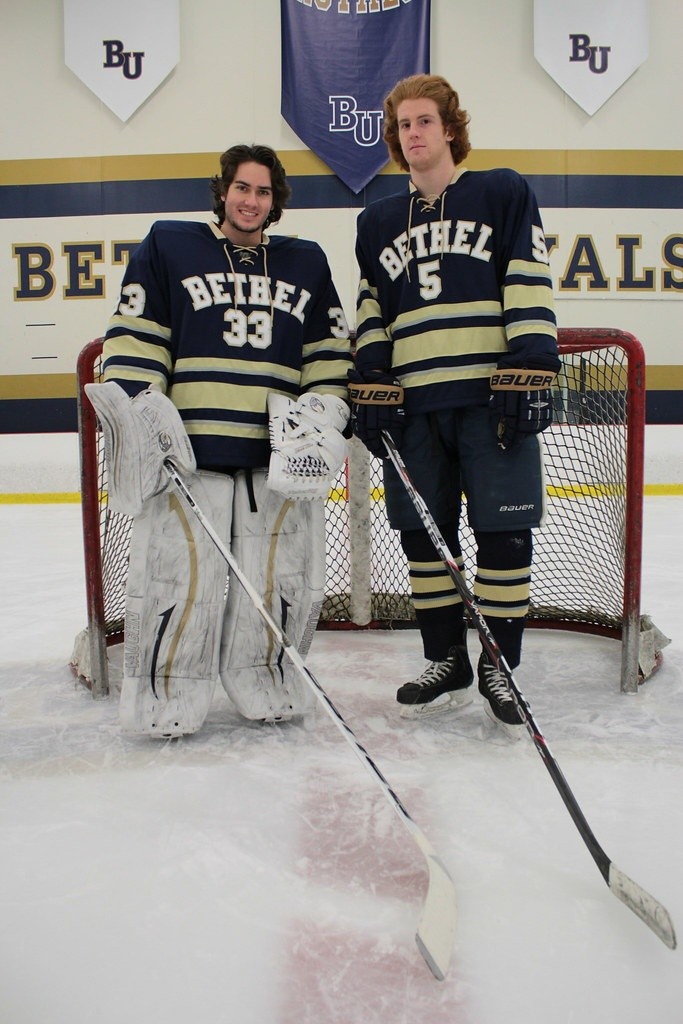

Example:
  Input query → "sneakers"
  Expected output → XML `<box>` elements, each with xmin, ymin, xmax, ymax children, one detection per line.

<box><xmin>478</xmin><ymin>654</ymin><xmax>526</xmax><ymax>739</ymax></box>
<box><xmin>397</xmin><ymin>645</ymin><xmax>474</xmax><ymax>719</ymax></box>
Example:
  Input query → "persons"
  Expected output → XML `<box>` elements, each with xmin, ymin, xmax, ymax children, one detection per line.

<box><xmin>348</xmin><ymin>74</ymin><xmax>560</xmax><ymax>740</ymax></box>
<box><xmin>85</xmin><ymin>146</ymin><xmax>352</xmax><ymax>739</ymax></box>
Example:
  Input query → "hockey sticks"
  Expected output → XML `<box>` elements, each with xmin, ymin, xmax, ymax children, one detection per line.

<box><xmin>382</xmin><ymin>424</ymin><xmax>678</xmax><ymax>953</ymax></box>
<box><xmin>160</xmin><ymin>458</ymin><xmax>462</xmax><ymax>984</ymax></box>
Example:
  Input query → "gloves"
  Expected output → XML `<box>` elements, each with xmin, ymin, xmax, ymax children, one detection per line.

<box><xmin>346</xmin><ymin>368</ymin><xmax>404</xmax><ymax>462</ymax></box>
<box><xmin>489</xmin><ymin>351</ymin><xmax>561</xmax><ymax>451</ymax></box>
